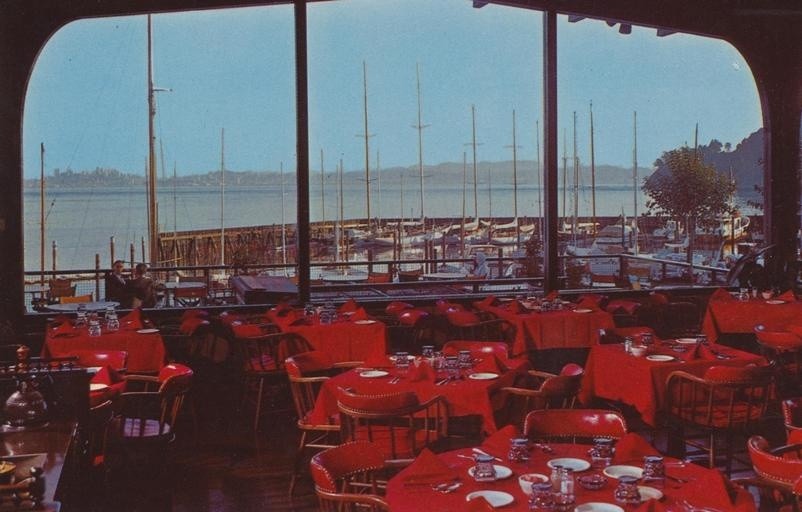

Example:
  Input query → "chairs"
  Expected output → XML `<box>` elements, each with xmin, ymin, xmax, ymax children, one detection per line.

<box><xmin>604</xmin><ymin>298</ymin><xmax>642</xmax><ymax>327</ymax></box>
<box><xmin>370</xmin><ymin>300</ymin><xmax>416</xmax><ymax>319</ymax></box>
<box><xmin>441</xmin><ymin>339</ymin><xmax>510</xmax><ymax>360</ymax></box>
<box><xmin>335</xmin><ymin>384</ymin><xmax>450</xmax><ymax>457</ymax></box>
<box><xmin>446</xmin><ymin>309</ymin><xmax>513</xmax><ymax>342</ymax></box>
<box><xmin>491</xmin><ymin>362</ymin><xmax>585</xmax><ymax>436</ymax></box>
<box><xmin>781</xmin><ymin>397</ymin><xmax>802</xmax><ymax>454</ymax></box>
<box><xmin>232</xmin><ymin>322</ymin><xmax>314</xmax><ymax>436</ymax></box>
<box><xmin>746</xmin><ymin>434</ymin><xmax>802</xmax><ymax>511</ymax></box>
<box><xmin>649</xmin><ymin>292</ymin><xmax>704</xmax><ymax>338</ymax></box>
<box><xmin>119</xmin><ymin>360</ymin><xmax>194</xmax><ymax>448</ymax></box>
<box><xmin>523</xmin><ymin>406</ymin><xmax>628</xmax><ymax>446</ymax></box>
<box><xmin>366</xmin><ymin>271</ymin><xmax>394</xmax><ymax>282</ymax></box>
<box><xmin>752</xmin><ymin>320</ymin><xmax>802</xmax><ymax>394</ymax></box>
<box><xmin>77</xmin><ymin>292</ymin><xmax>95</xmax><ymax>304</ymax></box>
<box><xmin>221</xmin><ymin>311</ymin><xmax>274</xmax><ymax>327</ymax></box>
<box><xmin>285</xmin><ymin>348</ymin><xmax>365</xmax><ymax>498</ymax></box>
<box><xmin>380</xmin><ymin>306</ymin><xmax>430</xmax><ymax>354</ymax></box>
<box><xmin>594</xmin><ymin>325</ymin><xmax>657</xmax><ymax>346</ymax></box>
<box><xmin>662</xmin><ymin>362</ymin><xmax>777</xmax><ymax>481</ymax></box>
<box><xmin>63</xmin><ymin>350</ymin><xmax>130</xmax><ymax>402</ymax></box>
<box><xmin>429</xmin><ymin>301</ymin><xmax>485</xmax><ymax>318</ymax></box>
<box><xmin>573</xmin><ymin>294</ymin><xmax>608</xmax><ymax>308</ymax></box>
<box><xmin>311</xmin><ymin>440</ymin><xmax>416</xmax><ymax>512</ymax></box>
<box><xmin>727</xmin><ymin>477</ymin><xmax>800</xmax><ymax>511</ymax></box>
<box><xmin>180</xmin><ymin>308</ymin><xmax>213</xmax><ymax>365</ymax></box>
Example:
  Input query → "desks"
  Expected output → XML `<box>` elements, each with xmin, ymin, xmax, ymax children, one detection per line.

<box><xmin>77</xmin><ymin>301</ymin><xmax>121</xmax><ymax>314</ymax></box>
<box><xmin>163</xmin><ymin>280</ymin><xmax>209</xmax><ymax>308</ymax></box>
<box><xmin>229</xmin><ymin>274</ymin><xmax>300</xmax><ymax>306</ymax></box>
<box><xmin>321</xmin><ymin>274</ymin><xmax>370</xmax><ymax>283</ymax></box>
<box><xmin>419</xmin><ymin>271</ymin><xmax>476</xmax><ymax>286</ymax></box>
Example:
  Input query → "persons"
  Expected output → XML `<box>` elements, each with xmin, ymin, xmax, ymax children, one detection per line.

<box><xmin>128</xmin><ymin>263</ymin><xmax>156</xmax><ymax>308</ymax></box>
<box><xmin>470</xmin><ymin>253</ymin><xmax>489</xmax><ymax>281</ymax></box>
<box><xmin>106</xmin><ymin>260</ymin><xmax>130</xmax><ymax>308</ymax></box>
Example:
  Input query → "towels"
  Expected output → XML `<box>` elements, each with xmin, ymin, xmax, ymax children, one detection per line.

<box><xmin>398</xmin><ymin>446</ymin><xmax>459</xmax><ymax>487</ymax></box>
<box><xmin>681</xmin><ymin>342</ymin><xmax>715</xmax><ymax>362</ymax></box>
<box><xmin>410</xmin><ymin>361</ymin><xmax>438</xmax><ymax>384</ymax></box>
<box><xmin>350</xmin><ymin>306</ymin><xmax>370</xmax><ymax>322</ymax></box>
<box><xmin>444</xmin><ymin>347</ymin><xmax>459</xmax><ymax>356</ymax></box>
<box><xmin>121</xmin><ymin>307</ymin><xmax>142</xmax><ymax>322</ymax></box>
<box><xmin>472</xmin><ymin>353</ymin><xmax>509</xmax><ymax>377</ymax></box>
<box><xmin>285</xmin><ymin>308</ymin><xmax>308</xmax><ymax>326</ymax></box>
<box><xmin>482</xmin><ymin>295</ymin><xmax>501</xmax><ymax>307</ymax></box>
<box><xmin>712</xmin><ymin>287</ymin><xmax>732</xmax><ymax>300</ymax></box>
<box><xmin>457</xmin><ymin>496</ymin><xmax>496</xmax><ymax>511</ymax></box>
<box><xmin>275</xmin><ymin>304</ymin><xmax>294</xmax><ymax>317</ymax></box>
<box><xmin>128</xmin><ymin>309</ymin><xmax>146</xmax><ymax>331</ymax></box>
<box><xmin>609</xmin><ymin>432</ymin><xmax>664</xmax><ymax>466</ymax></box>
<box><xmin>361</xmin><ymin>352</ymin><xmax>395</xmax><ymax>372</ymax></box>
<box><xmin>52</xmin><ymin>312</ymin><xmax>73</xmax><ymax>325</ymax></box>
<box><xmin>679</xmin><ymin>468</ymin><xmax>739</xmax><ymax>509</ymax></box>
<box><xmin>507</xmin><ymin>299</ymin><xmax>529</xmax><ymax>314</ymax></box>
<box><xmin>545</xmin><ymin>290</ymin><xmax>561</xmax><ymax>302</ymax></box>
<box><xmin>52</xmin><ymin>320</ymin><xmax>78</xmax><ymax>337</ymax></box>
<box><xmin>577</xmin><ymin>296</ymin><xmax>599</xmax><ymax>312</ymax></box>
<box><xmin>777</xmin><ymin>289</ymin><xmax>798</xmax><ymax>303</ymax></box>
<box><xmin>87</xmin><ymin>363</ymin><xmax>123</xmax><ymax>386</ymax></box>
<box><xmin>339</xmin><ymin>299</ymin><xmax>359</xmax><ymax>313</ymax></box>
<box><xmin>483</xmin><ymin>423</ymin><xmax>534</xmax><ymax>461</ymax></box>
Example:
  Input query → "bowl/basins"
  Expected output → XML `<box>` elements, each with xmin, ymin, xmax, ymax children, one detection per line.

<box><xmin>518</xmin><ymin>473</ymin><xmax>549</xmax><ymax>496</ymax></box>
<box><xmin>762</xmin><ymin>291</ymin><xmax>775</xmax><ymax>299</ymax></box>
<box><xmin>577</xmin><ymin>473</ymin><xmax>608</xmax><ymax>490</ymax></box>
<box><xmin>630</xmin><ymin>344</ymin><xmax>648</xmax><ymax>357</ymax></box>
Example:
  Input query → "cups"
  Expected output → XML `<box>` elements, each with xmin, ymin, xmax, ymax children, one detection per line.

<box><xmin>456</xmin><ymin>350</ymin><xmax>476</xmax><ymax>369</ymax></box>
<box><xmin>697</xmin><ymin>334</ymin><xmax>705</xmax><ymax>343</ymax></box>
<box><xmin>75</xmin><ymin>310</ymin><xmax>87</xmax><ymax>327</ymax></box>
<box><xmin>434</xmin><ymin>351</ymin><xmax>447</xmax><ymax>373</ymax></box>
<box><xmin>739</xmin><ymin>288</ymin><xmax>750</xmax><ymax>302</ymax></box>
<box><xmin>107</xmin><ymin>315</ymin><xmax>119</xmax><ymax>331</ymax></box>
<box><xmin>319</xmin><ymin>310</ymin><xmax>332</xmax><ymax>324</ymax></box>
<box><xmin>528</xmin><ymin>482</ymin><xmax>555</xmax><ymax>511</ymax></box>
<box><xmin>423</xmin><ymin>345</ymin><xmax>435</xmax><ymax>357</ymax></box>
<box><xmin>541</xmin><ymin>302</ymin><xmax>551</xmax><ymax>313</ymax></box>
<box><xmin>553</xmin><ymin>298</ymin><xmax>563</xmax><ymax>310</ymax></box>
<box><xmin>446</xmin><ymin>357</ymin><xmax>460</xmax><ymax>379</ymax></box>
<box><xmin>643</xmin><ymin>456</ymin><xmax>668</xmax><ymax>482</ymax></box>
<box><xmin>473</xmin><ymin>455</ymin><xmax>497</xmax><ymax>483</ymax></box>
<box><xmin>88</xmin><ymin>320</ymin><xmax>101</xmax><ymax>336</ymax></box>
<box><xmin>510</xmin><ymin>437</ymin><xmax>529</xmax><ymax>464</ymax></box>
<box><xmin>396</xmin><ymin>351</ymin><xmax>408</xmax><ymax>370</ymax></box>
<box><xmin>641</xmin><ymin>333</ymin><xmax>656</xmax><ymax>350</ymax></box>
<box><xmin>105</xmin><ymin>305</ymin><xmax>116</xmax><ymax>320</ymax></box>
<box><xmin>617</xmin><ymin>475</ymin><xmax>642</xmax><ymax>509</ymax></box>
<box><xmin>594</xmin><ymin>436</ymin><xmax>614</xmax><ymax>465</ymax></box>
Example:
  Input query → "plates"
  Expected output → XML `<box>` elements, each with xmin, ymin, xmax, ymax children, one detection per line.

<box><xmin>127</xmin><ymin>318</ymin><xmax>152</xmax><ymax>325</ymax></box>
<box><xmin>766</xmin><ymin>300</ymin><xmax>788</xmax><ymax>305</ymax></box>
<box><xmin>647</xmin><ymin>354</ymin><xmax>675</xmax><ymax>362</ymax></box>
<box><xmin>604</xmin><ymin>464</ymin><xmax>650</xmax><ymax>481</ymax></box>
<box><xmin>89</xmin><ymin>383</ymin><xmax>111</xmax><ymax>391</ymax></box>
<box><xmin>361</xmin><ymin>369</ymin><xmax>389</xmax><ymax>379</ymax></box>
<box><xmin>677</xmin><ymin>338</ymin><xmax>697</xmax><ymax>344</ymax></box>
<box><xmin>469</xmin><ymin>372</ymin><xmax>499</xmax><ymax>380</ymax></box>
<box><xmin>136</xmin><ymin>329</ymin><xmax>162</xmax><ymax>334</ymax></box>
<box><xmin>390</xmin><ymin>354</ymin><xmax>417</xmax><ymax>362</ymax></box>
<box><xmin>572</xmin><ymin>502</ymin><xmax>625</xmax><ymax>512</ymax></box>
<box><xmin>638</xmin><ymin>486</ymin><xmax>665</xmax><ymax>502</ymax></box>
<box><xmin>574</xmin><ymin>307</ymin><xmax>593</xmax><ymax>313</ymax></box>
<box><xmin>354</xmin><ymin>319</ymin><xmax>376</xmax><ymax>325</ymax></box>
<box><xmin>343</xmin><ymin>310</ymin><xmax>353</xmax><ymax>319</ymax></box>
<box><xmin>546</xmin><ymin>458</ymin><xmax>591</xmax><ymax>473</ymax></box>
<box><xmin>466</xmin><ymin>490</ymin><xmax>516</xmax><ymax>508</ymax></box>
<box><xmin>469</xmin><ymin>463</ymin><xmax>513</xmax><ymax>481</ymax></box>
